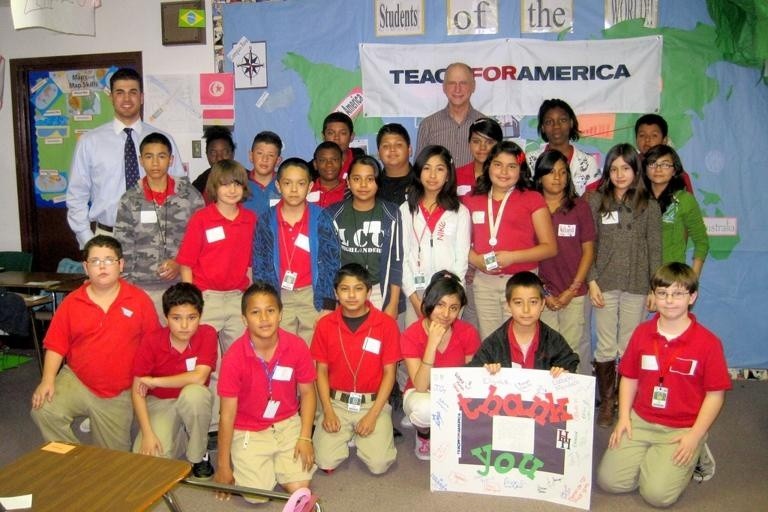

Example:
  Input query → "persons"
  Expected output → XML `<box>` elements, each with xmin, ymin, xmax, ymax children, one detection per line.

<box><xmin>596</xmin><ymin>263</ymin><xmax>734</xmax><ymax>507</ymax></box>
<box><xmin>305</xmin><ymin>142</ymin><xmax>349</xmax><ymax>210</ymax></box>
<box><xmin>396</xmin><ymin>146</ymin><xmax>471</xmax><ymax>428</ymax></box>
<box><xmin>189</xmin><ymin>126</ymin><xmax>250</xmax><ymax>209</ymax></box>
<box><xmin>532</xmin><ymin>148</ymin><xmax>597</xmax><ymax>375</ymax></box>
<box><xmin>632</xmin><ymin>114</ymin><xmax>694</xmax><ymax>246</ymax></box>
<box><xmin>251</xmin><ymin>157</ymin><xmax>342</xmax><ymax>439</ymax></box>
<box><xmin>585</xmin><ymin>143</ymin><xmax>662</xmax><ymax>428</ymax></box>
<box><xmin>132</xmin><ymin>282</ymin><xmax>218</xmax><ymax>481</ymax></box>
<box><xmin>641</xmin><ymin>143</ymin><xmax>710</xmax><ymax>281</ymax></box>
<box><xmin>211</xmin><ymin>284</ymin><xmax>317</xmax><ymax>503</ymax></box>
<box><xmin>30</xmin><ymin>235</ymin><xmax>158</xmax><ymax>454</ymax></box>
<box><xmin>463</xmin><ymin>140</ymin><xmax>558</xmax><ymax>345</ymax></box>
<box><xmin>176</xmin><ymin>158</ymin><xmax>258</xmax><ymax>451</ymax></box>
<box><xmin>451</xmin><ymin>117</ymin><xmax>504</xmax><ymax>203</ymax></box>
<box><xmin>313</xmin><ymin>155</ymin><xmax>404</xmax><ymax>444</ymax></box>
<box><xmin>309</xmin><ymin>263</ymin><xmax>404</xmax><ymax>476</ymax></box>
<box><xmin>66</xmin><ymin>67</ymin><xmax>185</xmax><ymax>254</ymax></box>
<box><xmin>373</xmin><ymin>123</ymin><xmax>417</xmax><ymax>207</ymax></box>
<box><xmin>112</xmin><ymin>133</ymin><xmax>205</xmax><ymax>329</ymax></box>
<box><xmin>464</xmin><ymin>270</ymin><xmax>580</xmax><ymax>378</ymax></box>
<box><xmin>307</xmin><ymin>112</ymin><xmax>365</xmax><ymax>183</ymax></box>
<box><xmin>239</xmin><ymin>131</ymin><xmax>283</xmax><ymax>217</ymax></box>
<box><xmin>524</xmin><ymin>98</ymin><xmax>603</xmax><ymax>201</ymax></box>
<box><xmin>400</xmin><ymin>270</ymin><xmax>481</xmax><ymax>461</ymax></box>
<box><xmin>415</xmin><ymin>61</ymin><xmax>489</xmax><ymax>170</ymax></box>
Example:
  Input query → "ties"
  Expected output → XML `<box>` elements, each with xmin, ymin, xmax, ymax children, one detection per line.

<box><xmin>122</xmin><ymin>126</ymin><xmax>140</xmax><ymax>192</ymax></box>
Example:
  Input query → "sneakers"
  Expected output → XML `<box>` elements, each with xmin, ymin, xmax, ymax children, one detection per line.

<box><xmin>411</xmin><ymin>431</ymin><xmax>431</xmax><ymax>463</ymax></box>
<box><xmin>693</xmin><ymin>434</ymin><xmax>717</xmax><ymax>486</ymax></box>
<box><xmin>188</xmin><ymin>452</ymin><xmax>216</xmax><ymax>482</ymax></box>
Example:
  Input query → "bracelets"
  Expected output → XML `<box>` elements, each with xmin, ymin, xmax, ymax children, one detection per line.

<box><xmin>570</xmin><ymin>285</ymin><xmax>579</xmax><ymax>295</ymax></box>
<box><xmin>543</xmin><ymin>288</ymin><xmax>551</xmax><ymax>296</ymax></box>
<box><xmin>297</xmin><ymin>437</ymin><xmax>313</xmax><ymax>442</ymax></box>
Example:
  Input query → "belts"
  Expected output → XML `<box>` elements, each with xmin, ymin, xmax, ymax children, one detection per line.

<box><xmin>97</xmin><ymin>223</ymin><xmax>113</xmax><ymax>232</ymax></box>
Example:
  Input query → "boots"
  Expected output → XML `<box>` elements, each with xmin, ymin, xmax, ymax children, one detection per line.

<box><xmin>591</xmin><ymin>358</ymin><xmax>619</xmax><ymax>430</ymax></box>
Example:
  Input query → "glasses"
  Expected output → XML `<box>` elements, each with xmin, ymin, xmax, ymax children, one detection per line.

<box><xmin>648</xmin><ymin>160</ymin><xmax>675</xmax><ymax>168</ymax></box>
<box><xmin>85</xmin><ymin>255</ymin><xmax>119</xmax><ymax>267</ymax></box>
<box><xmin>654</xmin><ymin>288</ymin><xmax>689</xmax><ymax>299</ymax></box>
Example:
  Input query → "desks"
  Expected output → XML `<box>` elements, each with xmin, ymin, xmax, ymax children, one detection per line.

<box><xmin>11</xmin><ymin>291</ymin><xmax>54</xmax><ymax>378</ymax></box>
<box><xmin>0</xmin><ymin>439</ymin><xmax>193</xmax><ymax>512</ymax></box>
<box><xmin>1</xmin><ymin>270</ymin><xmax>89</xmax><ymax>316</ymax></box>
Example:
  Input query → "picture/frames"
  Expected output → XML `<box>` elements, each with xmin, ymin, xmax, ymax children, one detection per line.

<box><xmin>232</xmin><ymin>40</ymin><xmax>268</xmax><ymax>91</ymax></box>
